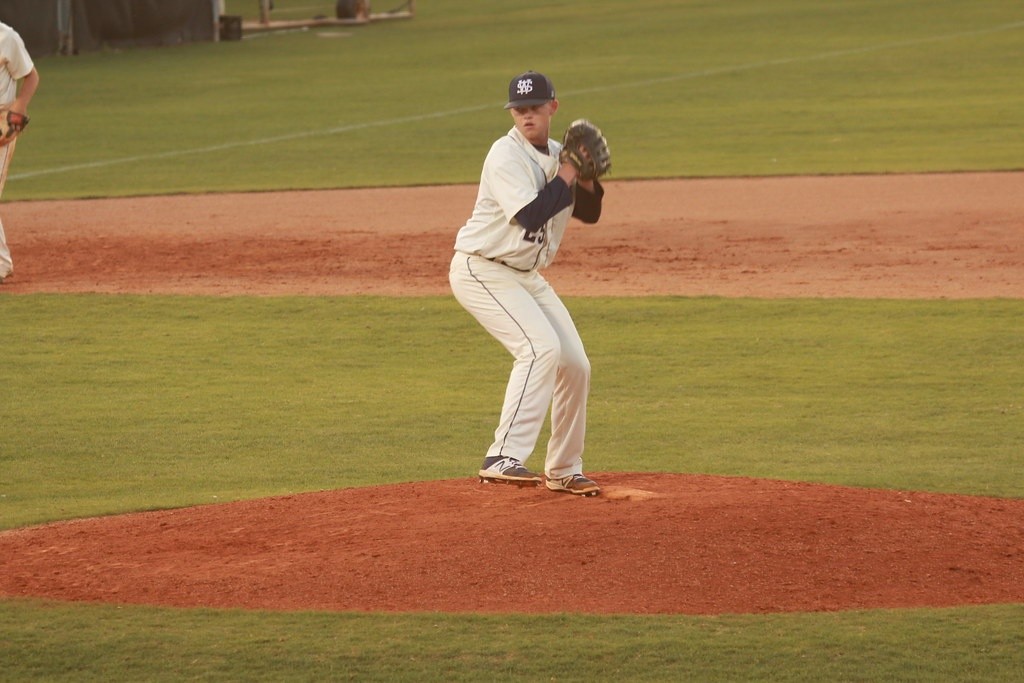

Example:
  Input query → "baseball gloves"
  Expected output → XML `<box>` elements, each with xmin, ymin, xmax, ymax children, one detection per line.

<box><xmin>560</xmin><ymin>118</ymin><xmax>612</xmax><ymax>181</ymax></box>
<box><xmin>0</xmin><ymin>106</ymin><xmax>34</xmax><ymax>150</ymax></box>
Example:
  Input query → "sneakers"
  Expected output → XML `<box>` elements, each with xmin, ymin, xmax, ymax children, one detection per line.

<box><xmin>545</xmin><ymin>474</ymin><xmax>601</xmax><ymax>498</ymax></box>
<box><xmin>479</xmin><ymin>456</ymin><xmax>543</xmax><ymax>488</ymax></box>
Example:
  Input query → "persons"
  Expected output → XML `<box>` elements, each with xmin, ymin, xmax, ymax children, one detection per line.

<box><xmin>449</xmin><ymin>70</ymin><xmax>610</xmax><ymax>496</ymax></box>
<box><xmin>0</xmin><ymin>23</ymin><xmax>39</xmax><ymax>281</ymax></box>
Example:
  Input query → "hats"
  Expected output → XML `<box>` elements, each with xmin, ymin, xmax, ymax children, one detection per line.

<box><xmin>504</xmin><ymin>68</ymin><xmax>556</xmax><ymax>110</ymax></box>
